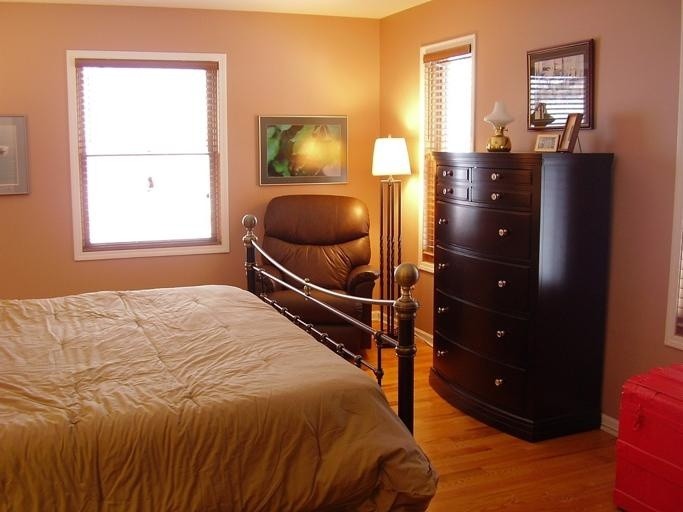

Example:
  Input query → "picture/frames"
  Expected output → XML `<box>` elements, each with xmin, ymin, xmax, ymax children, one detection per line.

<box><xmin>257</xmin><ymin>114</ymin><xmax>349</xmax><ymax>185</ymax></box>
<box><xmin>0</xmin><ymin>116</ymin><xmax>29</xmax><ymax>196</ymax></box>
<box><xmin>533</xmin><ymin>111</ymin><xmax>582</xmax><ymax>154</ymax></box>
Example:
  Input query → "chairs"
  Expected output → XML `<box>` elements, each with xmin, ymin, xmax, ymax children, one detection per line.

<box><xmin>255</xmin><ymin>194</ymin><xmax>380</xmax><ymax>356</ymax></box>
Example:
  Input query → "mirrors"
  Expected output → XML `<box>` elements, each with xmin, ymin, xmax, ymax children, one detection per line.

<box><xmin>525</xmin><ymin>37</ymin><xmax>595</xmax><ymax>131</ymax></box>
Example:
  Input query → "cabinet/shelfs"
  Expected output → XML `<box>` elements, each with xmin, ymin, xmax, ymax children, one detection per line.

<box><xmin>428</xmin><ymin>149</ymin><xmax>614</xmax><ymax>443</ymax></box>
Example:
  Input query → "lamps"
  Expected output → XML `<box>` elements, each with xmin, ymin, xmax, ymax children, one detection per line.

<box><xmin>370</xmin><ymin>138</ymin><xmax>412</xmax><ymax>348</ymax></box>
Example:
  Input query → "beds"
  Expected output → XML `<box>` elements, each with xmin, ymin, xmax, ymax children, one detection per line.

<box><xmin>0</xmin><ymin>214</ymin><xmax>438</xmax><ymax>511</ymax></box>
